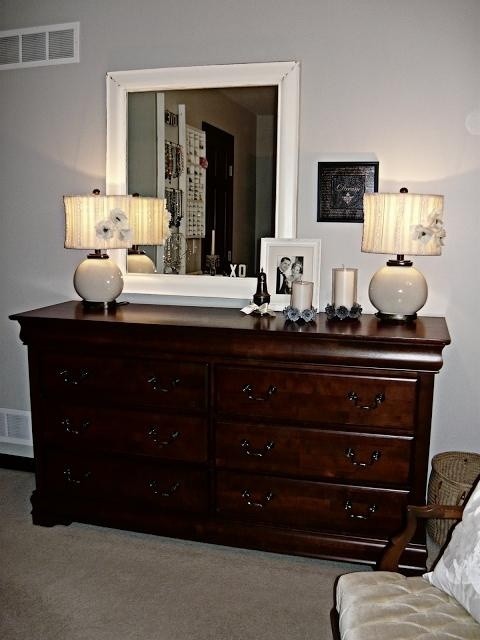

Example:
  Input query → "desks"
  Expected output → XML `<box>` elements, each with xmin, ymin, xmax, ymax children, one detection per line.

<box><xmin>6</xmin><ymin>300</ymin><xmax>452</xmax><ymax>575</ymax></box>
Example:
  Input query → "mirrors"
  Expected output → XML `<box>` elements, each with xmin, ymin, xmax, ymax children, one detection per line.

<box><xmin>107</xmin><ymin>60</ymin><xmax>303</xmax><ymax>310</ymax></box>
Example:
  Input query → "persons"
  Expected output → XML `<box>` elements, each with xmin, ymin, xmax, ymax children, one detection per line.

<box><xmin>276</xmin><ymin>257</ymin><xmax>292</xmax><ymax>294</ymax></box>
<box><xmin>287</xmin><ymin>261</ymin><xmax>303</xmax><ymax>294</ymax></box>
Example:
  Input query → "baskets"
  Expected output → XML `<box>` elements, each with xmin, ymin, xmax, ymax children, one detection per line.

<box><xmin>427</xmin><ymin>452</ymin><xmax>479</xmax><ymax>546</ymax></box>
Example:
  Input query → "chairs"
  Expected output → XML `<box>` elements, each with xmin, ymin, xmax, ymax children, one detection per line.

<box><xmin>329</xmin><ymin>502</ymin><xmax>480</xmax><ymax>639</ymax></box>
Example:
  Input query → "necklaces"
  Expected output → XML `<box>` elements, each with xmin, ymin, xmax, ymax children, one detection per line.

<box><xmin>165</xmin><ymin>143</ymin><xmax>184</xmax><ymax>228</ymax></box>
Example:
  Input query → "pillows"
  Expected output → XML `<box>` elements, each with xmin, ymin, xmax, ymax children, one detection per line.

<box><xmin>422</xmin><ymin>482</ymin><xmax>480</xmax><ymax>626</ymax></box>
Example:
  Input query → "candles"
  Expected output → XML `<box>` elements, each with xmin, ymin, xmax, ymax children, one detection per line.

<box><xmin>332</xmin><ymin>264</ymin><xmax>357</xmax><ymax>309</ymax></box>
<box><xmin>211</xmin><ymin>228</ymin><xmax>216</xmax><ymax>255</ymax></box>
<box><xmin>291</xmin><ymin>275</ymin><xmax>314</xmax><ymax>311</ymax></box>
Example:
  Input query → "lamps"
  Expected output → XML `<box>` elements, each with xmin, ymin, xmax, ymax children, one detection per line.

<box><xmin>360</xmin><ymin>187</ymin><xmax>446</xmax><ymax>319</ymax></box>
<box><xmin>62</xmin><ymin>194</ymin><xmax>133</xmax><ymax>312</ymax></box>
<box><xmin>127</xmin><ymin>197</ymin><xmax>171</xmax><ymax>275</ymax></box>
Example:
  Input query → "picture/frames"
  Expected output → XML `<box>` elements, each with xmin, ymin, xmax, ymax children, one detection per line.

<box><xmin>316</xmin><ymin>159</ymin><xmax>380</xmax><ymax>225</ymax></box>
<box><xmin>258</xmin><ymin>235</ymin><xmax>321</xmax><ymax>314</ymax></box>
